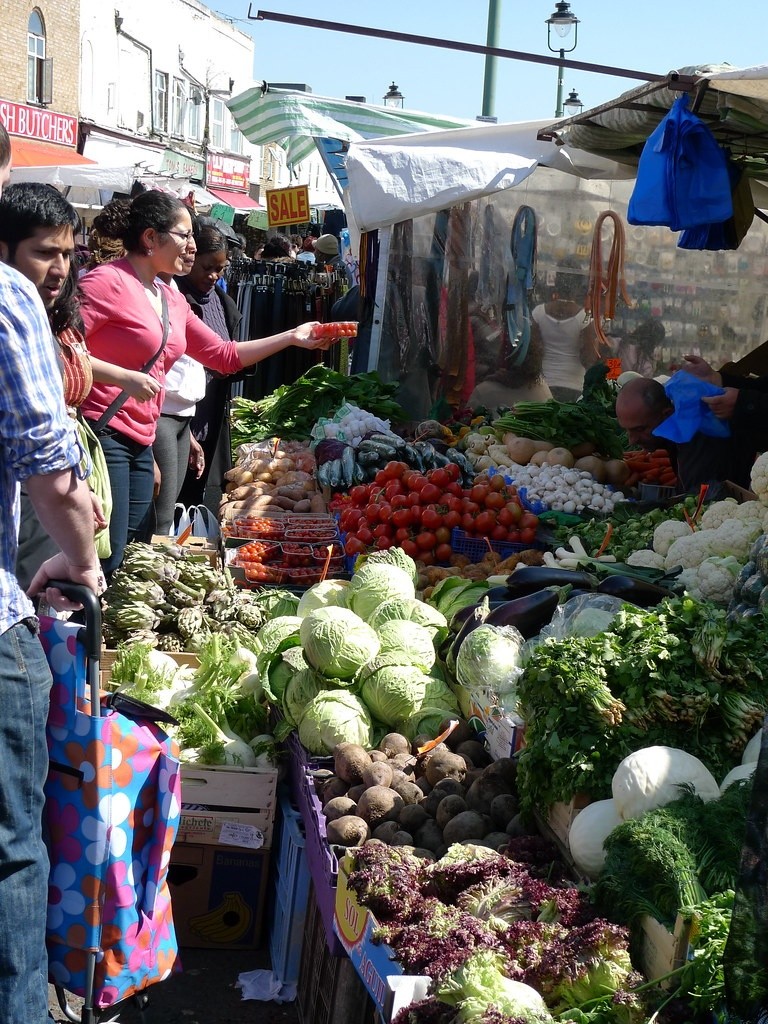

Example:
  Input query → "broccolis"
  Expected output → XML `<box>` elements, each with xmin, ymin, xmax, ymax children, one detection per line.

<box><xmin>750</xmin><ymin>451</ymin><xmax>768</xmax><ymax>507</ymax></box>
<box><xmin>623</xmin><ymin>501</ymin><xmax>768</xmax><ymax>604</ymax></box>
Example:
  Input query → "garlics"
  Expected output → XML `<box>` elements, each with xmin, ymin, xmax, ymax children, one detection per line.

<box><xmin>491</xmin><ymin>462</ymin><xmax>626</xmax><ymax>514</ymax></box>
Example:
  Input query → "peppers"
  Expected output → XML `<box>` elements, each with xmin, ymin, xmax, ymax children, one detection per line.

<box><xmin>442</xmin><ymin>405</ymin><xmax>489</xmax><ymax>432</ymax></box>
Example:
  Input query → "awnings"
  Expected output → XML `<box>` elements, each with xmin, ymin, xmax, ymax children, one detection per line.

<box><xmin>6</xmin><ymin>136</ymin><xmax>266</xmax><ymax>209</ymax></box>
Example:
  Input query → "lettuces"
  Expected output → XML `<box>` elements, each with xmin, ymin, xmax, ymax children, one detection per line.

<box><xmin>345</xmin><ymin>843</ymin><xmax>654</xmax><ymax>1024</ymax></box>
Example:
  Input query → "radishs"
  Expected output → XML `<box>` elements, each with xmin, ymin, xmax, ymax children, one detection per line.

<box><xmin>462</xmin><ymin>432</ymin><xmax>518</xmax><ymax>471</ymax></box>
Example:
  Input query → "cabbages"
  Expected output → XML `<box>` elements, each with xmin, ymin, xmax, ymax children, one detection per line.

<box><xmin>568</xmin><ymin>728</ymin><xmax>763</xmax><ymax>879</ymax></box>
<box><xmin>252</xmin><ymin>547</ymin><xmax>490</xmax><ymax>757</ymax></box>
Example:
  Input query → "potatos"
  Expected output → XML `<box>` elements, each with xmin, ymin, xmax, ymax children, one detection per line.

<box><xmin>223</xmin><ymin>439</ymin><xmax>325</xmax><ymax>520</ymax></box>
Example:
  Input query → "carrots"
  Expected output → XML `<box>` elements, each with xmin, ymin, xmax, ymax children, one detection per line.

<box><xmin>621</xmin><ymin>449</ymin><xmax>677</xmax><ymax>486</ymax></box>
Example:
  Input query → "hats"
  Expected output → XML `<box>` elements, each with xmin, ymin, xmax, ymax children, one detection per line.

<box><xmin>197</xmin><ymin>215</ymin><xmax>241</xmax><ymax>247</ymax></box>
<box><xmin>312</xmin><ymin>234</ymin><xmax>338</xmax><ymax>254</ymax></box>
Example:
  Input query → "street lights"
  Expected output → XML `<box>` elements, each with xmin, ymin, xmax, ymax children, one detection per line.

<box><xmin>545</xmin><ymin>0</ymin><xmax>581</xmax><ymax>119</ymax></box>
<box><xmin>563</xmin><ymin>87</ymin><xmax>587</xmax><ymax>119</ymax></box>
<box><xmin>382</xmin><ymin>80</ymin><xmax>405</xmax><ymax>109</ymax></box>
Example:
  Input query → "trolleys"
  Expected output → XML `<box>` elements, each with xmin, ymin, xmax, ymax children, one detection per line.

<box><xmin>18</xmin><ymin>578</ymin><xmax>182</xmax><ymax>1023</ymax></box>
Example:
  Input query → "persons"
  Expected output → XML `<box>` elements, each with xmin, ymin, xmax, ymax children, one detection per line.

<box><xmin>0</xmin><ymin>120</ymin><xmax>100</xmax><ymax>1023</ymax></box>
<box><xmin>529</xmin><ymin>256</ymin><xmax>593</xmax><ymax>404</ymax></box>
<box><xmin>616</xmin><ymin>317</ymin><xmax>666</xmax><ymax>379</ymax></box>
<box><xmin>0</xmin><ymin>181</ymin><xmax>398</xmax><ymax>631</ymax></box>
<box><xmin>667</xmin><ymin>339</ymin><xmax>768</xmax><ymax>492</ymax></box>
<box><xmin>464</xmin><ymin>313</ymin><xmax>554</xmax><ymax>419</ymax></box>
<box><xmin>616</xmin><ymin>378</ymin><xmax>722</xmax><ymax>506</ymax></box>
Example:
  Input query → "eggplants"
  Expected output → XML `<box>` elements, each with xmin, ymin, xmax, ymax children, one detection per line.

<box><xmin>319</xmin><ymin>433</ymin><xmax>474</xmax><ymax>487</ymax></box>
<box><xmin>436</xmin><ymin>565</ymin><xmax>684</xmax><ymax>680</ymax></box>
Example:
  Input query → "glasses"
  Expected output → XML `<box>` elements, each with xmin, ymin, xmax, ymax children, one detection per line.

<box><xmin>158</xmin><ymin>231</ymin><xmax>194</xmax><ymax>240</ymax></box>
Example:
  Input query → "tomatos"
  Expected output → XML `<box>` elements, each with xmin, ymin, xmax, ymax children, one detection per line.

<box><xmin>327</xmin><ymin>460</ymin><xmax>538</xmax><ymax>568</ymax></box>
<box><xmin>221</xmin><ymin>513</ymin><xmax>347</xmax><ymax>586</ymax></box>
<box><xmin>313</xmin><ymin>321</ymin><xmax>358</xmax><ymax>339</ymax></box>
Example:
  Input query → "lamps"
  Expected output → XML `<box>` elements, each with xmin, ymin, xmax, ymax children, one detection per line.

<box><xmin>545</xmin><ymin>0</ymin><xmax>581</xmax><ymax>57</ymax></box>
<box><xmin>382</xmin><ymin>81</ymin><xmax>405</xmax><ymax>109</ymax></box>
<box><xmin>562</xmin><ymin>87</ymin><xmax>584</xmax><ymax>118</ymax></box>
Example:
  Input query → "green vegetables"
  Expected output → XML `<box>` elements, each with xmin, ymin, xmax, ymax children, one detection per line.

<box><xmin>491</xmin><ymin>362</ymin><xmax>640</xmax><ymax>459</ymax></box>
<box><xmin>589</xmin><ymin>782</ymin><xmax>768</xmax><ymax>1024</ymax></box>
<box><xmin>230</xmin><ymin>363</ymin><xmax>405</xmax><ymax>460</ymax></box>
<box><xmin>514</xmin><ymin>597</ymin><xmax>766</xmax><ymax>820</ymax></box>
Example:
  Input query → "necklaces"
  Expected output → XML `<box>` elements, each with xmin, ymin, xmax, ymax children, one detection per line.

<box><xmin>556</xmin><ymin>299</ymin><xmax>576</xmax><ymax>304</ymax></box>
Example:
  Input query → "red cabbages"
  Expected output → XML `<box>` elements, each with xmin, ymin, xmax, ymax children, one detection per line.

<box><xmin>358</xmin><ymin>430</ymin><xmax>387</xmax><ymax>445</ymax></box>
<box><xmin>315</xmin><ymin>438</ymin><xmax>352</xmax><ymax>469</ymax></box>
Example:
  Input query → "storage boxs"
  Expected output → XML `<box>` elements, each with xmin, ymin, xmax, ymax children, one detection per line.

<box><xmin>168</xmin><ymin>842</ymin><xmax>271</xmax><ymax>951</ymax></box>
<box><xmin>266</xmin><ymin>774</ymin><xmax>313</xmax><ymax>987</ymax></box>
<box><xmin>293</xmin><ymin>874</ymin><xmax>373</xmax><ymax>1024</ymax></box>
<box><xmin>231</xmin><ymin>508</ymin><xmax>349</xmax><ymax>586</ymax></box>
<box><xmin>333</xmin><ymin>511</ymin><xmax>360</xmax><ymax>576</ymax></box>
<box><xmin>456</xmin><ymin>678</ymin><xmax>529</xmax><ymax>762</ymax></box>
<box><xmin>638</xmin><ymin>911</ymin><xmax>688</xmax><ymax>993</ymax></box>
<box><xmin>300</xmin><ymin>768</ymin><xmax>356</xmax><ymax>958</ymax></box>
<box><xmin>332</xmin><ymin>855</ymin><xmax>409</xmax><ymax>1011</ymax></box>
<box><xmin>666</xmin><ymin>479</ymin><xmax>760</xmax><ymax>506</ymax></box>
<box><xmin>638</xmin><ymin>480</ymin><xmax>677</xmax><ymax>501</ymax></box>
<box><xmin>100</xmin><ymin>635</ymin><xmax>202</xmax><ymax>692</ymax></box>
<box><xmin>290</xmin><ymin>731</ymin><xmax>336</xmax><ymax>816</ymax></box>
<box><xmin>488</xmin><ymin>464</ymin><xmax>637</xmax><ymax>517</ymax></box>
<box><xmin>311</xmin><ymin>322</ymin><xmax>359</xmax><ymax>339</ymax></box>
<box><xmin>174</xmin><ymin>761</ymin><xmax>279</xmax><ymax>852</ymax></box>
<box><xmin>445</xmin><ymin>527</ymin><xmax>529</xmax><ymax>568</ymax></box>
<box><xmin>519</xmin><ymin>732</ymin><xmax>594</xmax><ymax>848</ymax></box>
